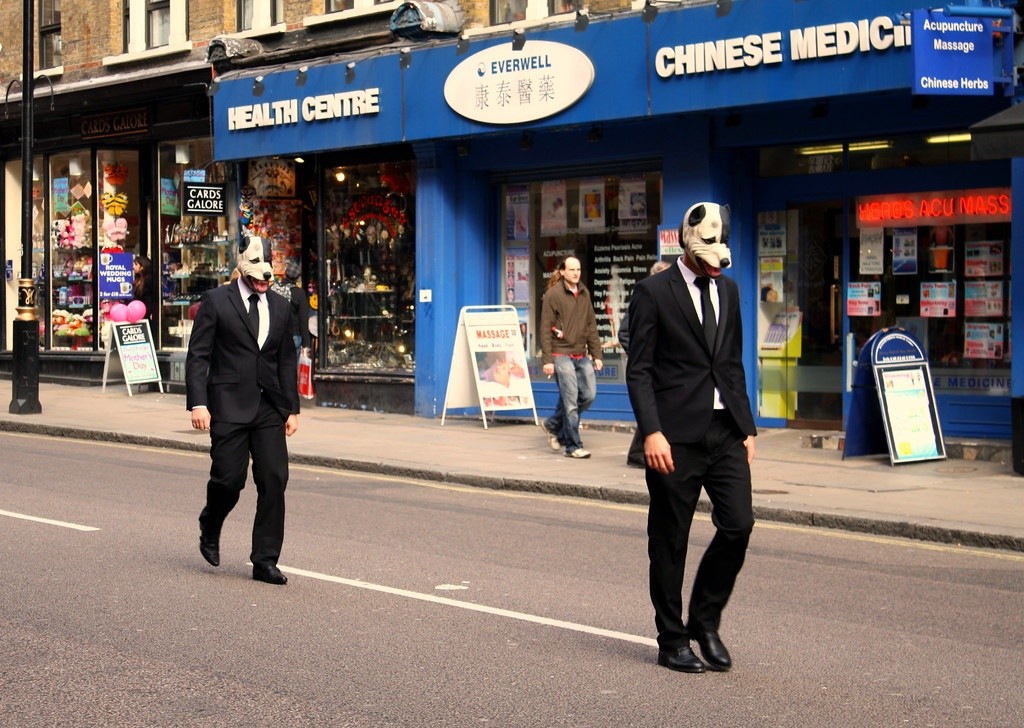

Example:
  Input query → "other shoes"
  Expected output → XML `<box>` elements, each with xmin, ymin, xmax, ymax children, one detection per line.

<box><xmin>541</xmin><ymin>421</ymin><xmax>560</xmax><ymax>451</ymax></box>
<box><xmin>564</xmin><ymin>448</ymin><xmax>591</xmax><ymax>458</ymax></box>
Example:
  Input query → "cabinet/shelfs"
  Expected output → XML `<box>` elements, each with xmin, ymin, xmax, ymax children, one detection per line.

<box><xmin>33</xmin><ymin>133</ymin><xmax>416</xmax><ymax>375</ymax></box>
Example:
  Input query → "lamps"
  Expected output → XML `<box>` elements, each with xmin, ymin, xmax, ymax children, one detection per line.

<box><xmin>205</xmin><ymin>0</ymin><xmax>730</xmax><ymax>93</ymax></box>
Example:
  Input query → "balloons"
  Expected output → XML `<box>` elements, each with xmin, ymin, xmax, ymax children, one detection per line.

<box><xmin>110</xmin><ymin>304</ymin><xmax>128</xmax><ymax>322</ymax></box>
<box><xmin>125</xmin><ymin>300</ymin><xmax>146</xmax><ymax>324</ymax></box>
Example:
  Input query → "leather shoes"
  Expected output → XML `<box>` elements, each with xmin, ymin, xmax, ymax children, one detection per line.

<box><xmin>685</xmin><ymin>625</ymin><xmax>731</xmax><ymax>669</ymax></box>
<box><xmin>658</xmin><ymin>646</ymin><xmax>706</xmax><ymax>673</ymax></box>
<box><xmin>199</xmin><ymin>536</ymin><xmax>220</xmax><ymax>567</ymax></box>
<box><xmin>253</xmin><ymin>564</ymin><xmax>288</xmax><ymax>584</ymax></box>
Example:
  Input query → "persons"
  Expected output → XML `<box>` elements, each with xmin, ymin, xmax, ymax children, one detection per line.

<box><xmin>585</xmin><ymin>193</ymin><xmax>602</xmax><ymax>218</ymax></box>
<box><xmin>479</xmin><ymin>357</ymin><xmax>525</xmax><ymax>387</ymax></box>
<box><xmin>119</xmin><ymin>256</ymin><xmax>159</xmax><ymax>350</ymax></box>
<box><xmin>761</xmin><ymin>286</ymin><xmax>778</xmax><ymax>303</ymax></box>
<box><xmin>185</xmin><ymin>236</ymin><xmax>301</xmax><ymax>584</ymax></box>
<box><xmin>519</xmin><ymin>322</ymin><xmax>527</xmax><ymax>352</ymax></box>
<box><xmin>507</xmin><ymin>262</ymin><xmax>514</xmax><ymax>301</ymax></box>
<box><xmin>546</xmin><ymin>197</ymin><xmax>566</xmax><ymax>220</ymax></box>
<box><xmin>539</xmin><ymin>256</ymin><xmax>603</xmax><ymax>459</ymax></box>
<box><xmin>625</xmin><ymin>201</ymin><xmax>759</xmax><ymax>674</ymax></box>
<box><xmin>281</xmin><ymin>263</ymin><xmax>310</xmax><ymax>436</ymax></box>
<box><xmin>542</xmin><ymin>263</ymin><xmax>563</xmax><ymax>300</ymax></box>
<box><xmin>618</xmin><ymin>261</ymin><xmax>673</xmax><ymax>468</ymax></box>
<box><xmin>601</xmin><ymin>265</ymin><xmax>625</xmax><ymax>348</ymax></box>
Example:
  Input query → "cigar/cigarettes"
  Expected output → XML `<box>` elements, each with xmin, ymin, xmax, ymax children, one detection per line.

<box><xmin>548</xmin><ymin>374</ymin><xmax>551</xmax><ymax>379</ymax></box>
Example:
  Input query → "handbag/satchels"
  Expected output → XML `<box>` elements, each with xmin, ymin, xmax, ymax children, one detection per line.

<box><xmin>297</xmin><ymin>348</ymin><xmax>314</xmax><ymax>400</ymax></box>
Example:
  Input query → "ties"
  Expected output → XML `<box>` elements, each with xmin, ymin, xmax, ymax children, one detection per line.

<box><xmin>248</xmin><ymin>294</ymin><xmax>259</xmax><ymax>339</ymax></box>
<box><xmin>694</xmin><ymin>277</ymin><xmax>718</xmax><ymax>357</ymax></box>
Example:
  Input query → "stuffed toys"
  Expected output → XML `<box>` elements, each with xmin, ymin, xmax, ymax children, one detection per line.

<box><xmin>105</xmin><ymin>162</ymin><xmax>129</xmax><ymax>185</ymax></box>
<box><xmin>101</xmin><ymin>192</ymin><xmax>130</xmax><ymax>242</ymax></box>
<box><xmin>51</xmin><ymin>209</ymin><xmax>92</xmax><ymax>251</ymax></box>
<box><xmin>52</xmin><ymin>308</ymin><xmax>93</xmax><ymax>337</ymax></box>
